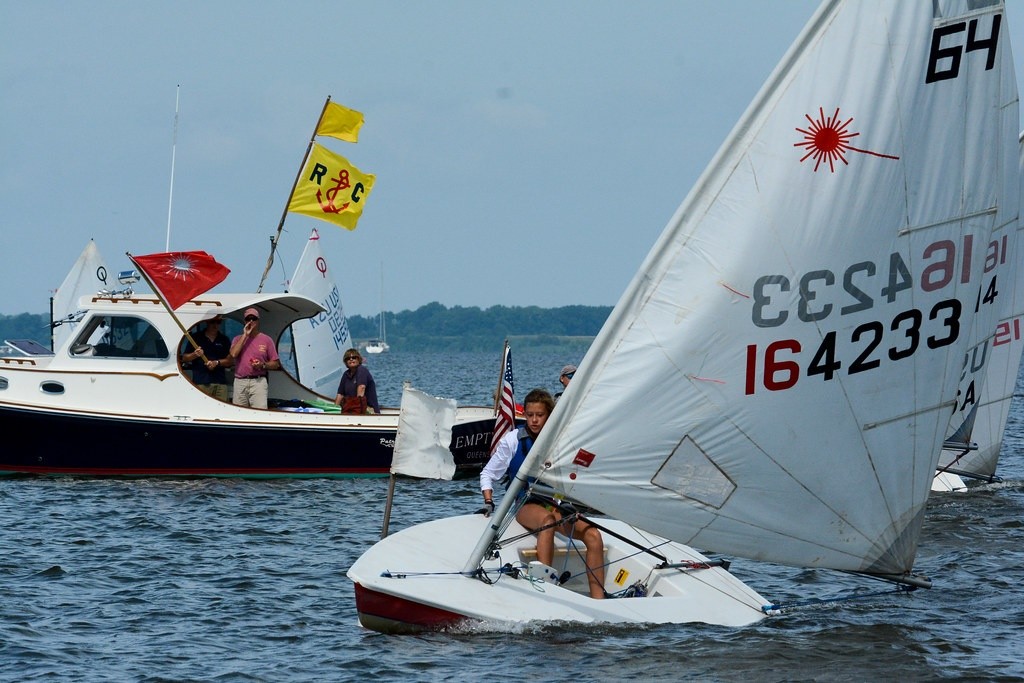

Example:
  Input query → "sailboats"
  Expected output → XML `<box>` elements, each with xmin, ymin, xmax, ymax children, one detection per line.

<box><xmin>926</xmin><ymin>103</ymin><xmax>1023</xmax><ymax>498</ymax></box>
<box><xmin>341</xmin><ymin>0</ymin><xmax>1015</xmax><ymax>641</ymax></box>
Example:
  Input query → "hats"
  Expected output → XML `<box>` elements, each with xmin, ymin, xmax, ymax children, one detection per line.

<box><xmin>244</xmin><ymin>308</ymin><xmax>259</xmax><ymax>319</ymax></box>
<box><xmin>560</xmin><ymin>365</ymin><xmax>577</xmax><ymax>376</ymax></box>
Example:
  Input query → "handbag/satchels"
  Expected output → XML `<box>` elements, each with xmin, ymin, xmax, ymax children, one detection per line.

<box><xmin>339</xmin><ymin>395</ymin><xmax>367</xmax><ymax>415</ymax></box>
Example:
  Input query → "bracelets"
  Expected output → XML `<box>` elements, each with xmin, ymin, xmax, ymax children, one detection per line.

<box><xmin>485</xmin><ymin>499</ymin><xmax>493</xmax><ymax>502</ymax></box>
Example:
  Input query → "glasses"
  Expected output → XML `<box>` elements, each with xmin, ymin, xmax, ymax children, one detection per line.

<box><xmin>246</xmin><ymin>316</ymin><xmax>257</xmax><ymax>321</ymax></box>
<box><xmin>566</xmin><ymin>374</ymin><xmax>574</xmax><ymax>379</ymax></box>
<box><xmin>209</xmin><ymin>319</ymin><xmax>222</xmax><ymax>324</ymax></box>
<box><xmin>346</xmin><ymin>356</ymin><xmax>357</xmax><ymax>360</ymax></box>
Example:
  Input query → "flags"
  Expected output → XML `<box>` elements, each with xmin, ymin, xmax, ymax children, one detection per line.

<box><xmin>287</xmin><ymin>140</ymin><xmax>377</xmax><ymax>231</ymax></box>
<box><xmin>489</xmin><ymin>343</ymin><xmax>516</xmax><ymax>459</ymax></box>
<box><xmin>390</xmin><ymin>385</ymin><xmax>458</xmax><ymax>480</ymax></box>
<box><xmin>316</xmin><ymin>101</ymin><xmax>364</xmax><ymax>143</ymax></box>
<box><xmin>131</xmin><ymin>250</ymin><xmax>231</xmax><ymax>311</ymax></box>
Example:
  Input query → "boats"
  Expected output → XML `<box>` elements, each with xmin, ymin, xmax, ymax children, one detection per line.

<box><xmin>366</xmin><ymin>281</ymin><xmax>389</xmax><ymax>354</ymax></box>
<box><xmin>1</xmin><ymin>262</ymin><xmax>526</xmax><ymax>484</ymax></box>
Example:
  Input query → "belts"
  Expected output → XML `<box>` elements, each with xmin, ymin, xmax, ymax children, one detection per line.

<box><xmin>243</xmin><ymin>374</ymin><xmax>264</xmax><ymax>379</ymax></box>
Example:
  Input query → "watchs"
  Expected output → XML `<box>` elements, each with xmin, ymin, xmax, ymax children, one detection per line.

<box><xmin>262</xmin><ymin>363</ymin><xmax>266</xmax><ymax>368</ymax></box>
<box><xmin>217</xmin><ymin>360</ymin><xmax>220</xmax><ymax>366</ymax></box>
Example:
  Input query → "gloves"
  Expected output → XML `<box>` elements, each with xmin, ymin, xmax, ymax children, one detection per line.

<box><xmin>474</xmin><ymin>501</ymin><xmax>495</xmax><ymax>517</ymax></box>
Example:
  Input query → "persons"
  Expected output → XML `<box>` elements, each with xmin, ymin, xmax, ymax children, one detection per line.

<box><xmin>335</xmin><ymin>348</ymin><xmax>381</xmax><ymax>414</ymax></box>
<box><xmin>553</xmin><ymin>364</ymin><xmax>578</xmax><ymax>406</ymax></box>
<box><xmin>472</xmin><ymin>388</ymin><xmax>607</xmax><ymax>600</ymax></box>
<box><xmin>182</xmin><ymin>315</ymin><xmax>233</xmax><ymax>404</ymax></box>
<box><xmin>229</xmin><ymin>308</ymin><xmax>280</xmax><ymax>410</ymax></box>
